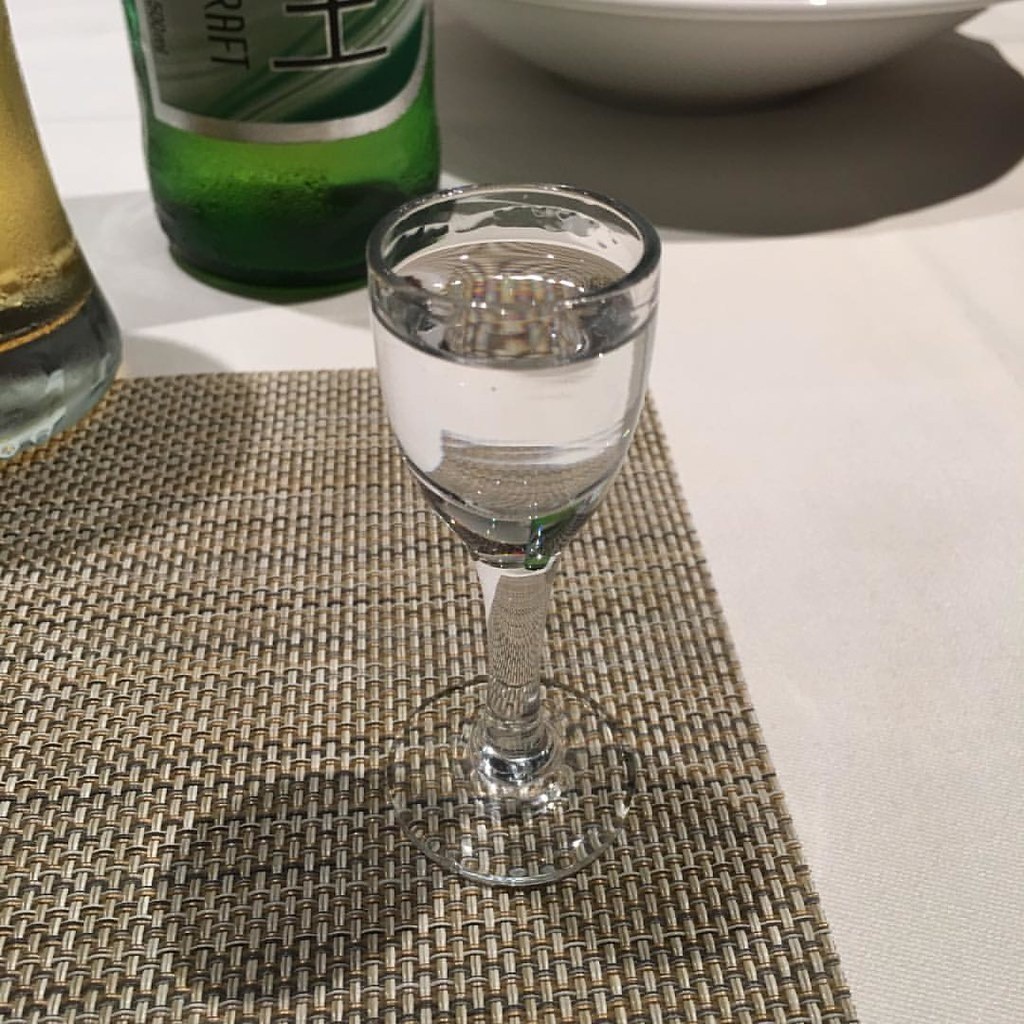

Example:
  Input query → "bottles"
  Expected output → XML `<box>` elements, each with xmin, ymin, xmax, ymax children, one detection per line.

<box><xmin>121</xmin><ymin>1</ymin><xmax>447</xmax><ymax>284</ymax></box>
<box><xmin>1</xmin><ymin>0</ymin><xmax>126</xmax><ymax>459</ymax></box>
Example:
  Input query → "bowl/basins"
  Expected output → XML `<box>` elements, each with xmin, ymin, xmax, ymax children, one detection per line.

<box><xmin>442</xmin><ymin>0</ymin><xmax>1013</xmax><ymax>113</ymax></box>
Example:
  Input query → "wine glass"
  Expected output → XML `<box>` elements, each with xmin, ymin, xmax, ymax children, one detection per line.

<box><xmin>366</xmin><ymin>183</ymin><xmax>665</xmax><ymax>890</ymax></box>
